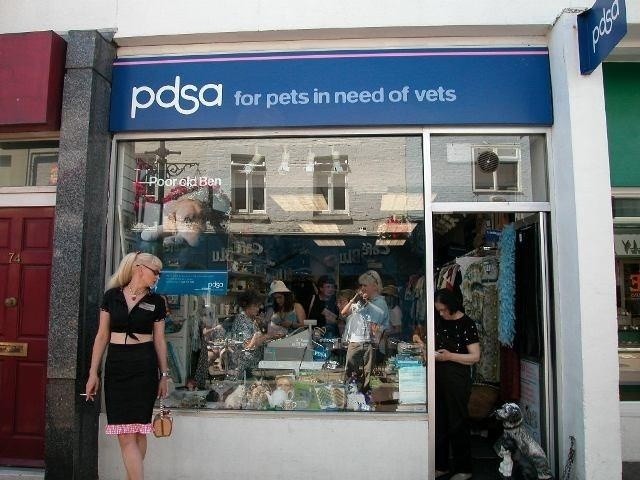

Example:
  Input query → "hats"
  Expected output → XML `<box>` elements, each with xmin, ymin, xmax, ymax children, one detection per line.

<box><xmin>268</xmin><ymin>281</ymin><xmax>291</xmax><ymax>297</ymax></box>
<box><xmin>381</xmin><ymin>285</ymin><xmax>400</xmax><ymax>299</ymax></box>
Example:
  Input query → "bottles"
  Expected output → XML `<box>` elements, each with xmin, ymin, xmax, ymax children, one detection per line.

<box><xmin>217</xmin><ymin>297</ymin><xmax>244</xmax><ymax>316</ymax></box>
<box><xmin>234</xmin><ymin>241</ymin><xmax>242</xmax><ymax>253</ymax></box>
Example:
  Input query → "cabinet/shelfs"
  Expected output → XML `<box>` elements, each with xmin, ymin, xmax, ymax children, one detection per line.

<box><xmin>162</xmin><ymin>253</ymin><xmax>292</xmax><ymax>388</ymax></box>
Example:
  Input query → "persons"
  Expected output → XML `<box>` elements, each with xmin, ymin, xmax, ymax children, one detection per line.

<box><xmin>413</xmin><ymin>288</ymin><xmax>480</xmax><ymax>480</ymax></box>
<box><xmin>86</xmin><ymin>251</ymin><xmax>167</xmax><ymax>480</ymax></box>
<box><xmin>204</xmin><ymin>270</ymin><xmax>403</xmax><ymax>391</ymax></box>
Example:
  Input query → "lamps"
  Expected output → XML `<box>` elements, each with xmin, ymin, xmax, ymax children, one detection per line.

<box><xmin>248</xmin><ymin>143</ymin><xmax>345</xmax><ymax>175</ymax></box>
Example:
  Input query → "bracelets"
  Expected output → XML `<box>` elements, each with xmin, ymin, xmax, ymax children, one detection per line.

<box><xmin>159</xmin><ymin>372</ymin><xmax>170</xmax><ymax>377</ymax></box>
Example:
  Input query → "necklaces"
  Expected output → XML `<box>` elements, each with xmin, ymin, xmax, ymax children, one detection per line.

<box><xmin>129</xmin><ymin>284</ymin><xmax>146</xmax><ymax>302</ymax></box>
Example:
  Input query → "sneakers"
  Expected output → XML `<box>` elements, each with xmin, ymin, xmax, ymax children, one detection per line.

<box><xmin>450</xmin><ymin>472</ymin><xmax>472</xmax><ymax>480</ymax></box>
<box><xmin>435</xmin><ymin>468</ymin><xmax>449</xmax><ymax>478</ymax></box>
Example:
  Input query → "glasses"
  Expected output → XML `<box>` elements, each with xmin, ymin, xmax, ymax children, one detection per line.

<box><xmin>136</xmin><ymin>264</ymin><xmax>161</xmax><ymax>277</ymax></box>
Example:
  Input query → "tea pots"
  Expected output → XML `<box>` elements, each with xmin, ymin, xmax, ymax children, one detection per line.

<box><xmin>263</xmin><ymin>387</ymin><xmax>295</xmax><ymax>408</ymax></box>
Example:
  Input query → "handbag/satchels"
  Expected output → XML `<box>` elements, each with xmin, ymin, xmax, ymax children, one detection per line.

<box><xmin>153</xmin><ymin>395</ymin><xmax>174</xmax><ymax>438</ymax></box>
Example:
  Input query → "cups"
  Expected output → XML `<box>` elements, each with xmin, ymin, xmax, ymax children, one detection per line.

<box><xmin>235</xmin><ymin>279</ymin><xmax>247</xmax><ymax>290</ymax></box>
<box><xmin>282</xmin><ymin>399</ymin><xmax>297</xmax><ymax>410</ymax></box>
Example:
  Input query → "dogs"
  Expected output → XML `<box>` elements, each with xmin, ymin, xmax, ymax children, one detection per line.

<box><xmin>140</xmin><ymin>182</ymin><xmax>231</xmax><ymax>250</ymax></box>
<box><xmin>492</xmin><ymin>402</ymin><xmax>551</xmax><ymax>480</ymax></box>
